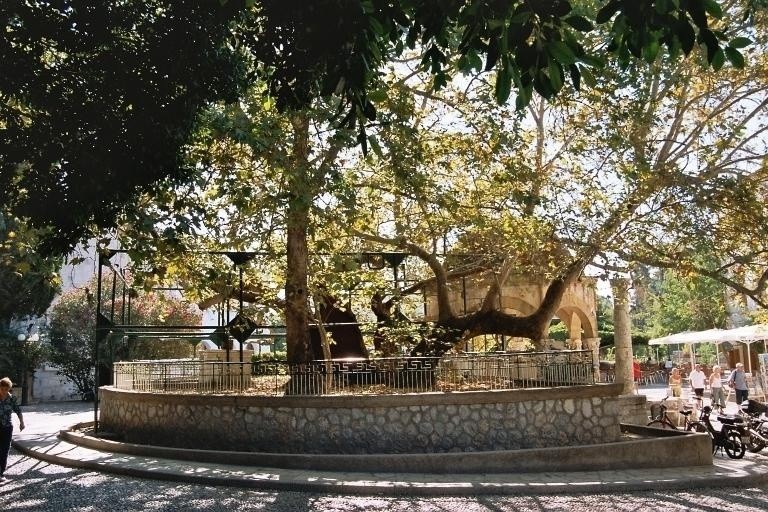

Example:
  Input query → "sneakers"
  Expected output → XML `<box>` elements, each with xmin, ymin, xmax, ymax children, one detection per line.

<box><xmin>0</xmin><ymin>474</ymin><xmax>6</xmax><ymax>481</ymax></box>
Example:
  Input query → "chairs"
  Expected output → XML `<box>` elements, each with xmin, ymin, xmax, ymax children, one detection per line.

<box><xmin>641</xmin><ymin>364</ymin><xmax>667</xmax><ymax>387</ymax></box>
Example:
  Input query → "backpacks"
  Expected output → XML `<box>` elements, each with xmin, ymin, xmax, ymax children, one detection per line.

<box><xmin>728</xmin><ymin>381</ymin><xmax>736</xmax><ymax>387</ymax></box>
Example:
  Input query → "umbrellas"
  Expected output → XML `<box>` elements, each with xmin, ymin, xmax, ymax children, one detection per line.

<box><xmin>647</xmin><ymin>325</ymin><xmax>768</xmax><ymax>374</ymax></box>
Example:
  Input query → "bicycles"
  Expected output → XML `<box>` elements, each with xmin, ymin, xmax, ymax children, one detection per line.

<box><xmin>647</xmin><ymin>397</ymin><xmax>714</xmax><ymax>455</ymax></box>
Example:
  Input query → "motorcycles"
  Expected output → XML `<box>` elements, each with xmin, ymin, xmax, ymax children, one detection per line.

<box><xmin>695</xmin><ymin>397</ymin><xmax>768</xmax><ymax>457</ymax></box>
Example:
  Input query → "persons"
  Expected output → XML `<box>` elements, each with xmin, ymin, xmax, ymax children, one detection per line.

<box><xmin>669</xmin><ymin>367</ymin><xmax>682</xmax><ymax>398</ymax></box>
<box><xmin>0</xmin><ymin>376</ymin><xmax>26</xmax><ymax>481</ymax></box>
<box><xmin>728</xmin><ymin>362</ymin><xmax>750</xmax><ymax>411</ymax></box>
<box><xmin>646</xmin><ymin>357</ymin><xmax>653</xmax><ymax>364</ymax></box>
<box><xmin>663</xmin><ymin>359</ymin><xmax>673</xmax><ymax>369</ymax></box>
<box><xmin>689</xmin><ymin>364</ymin><xmax>707</xmax><ymax>408</ymax></box>
<box><xmin>708</xmin><ymin>365</ymin><xmax>728</xmax><ymax>416</ymax></box>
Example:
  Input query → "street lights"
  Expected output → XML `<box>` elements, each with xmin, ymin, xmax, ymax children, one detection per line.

<box><xmin>17</xmin><ymin>324</ymin><xmax>39</xmax><ymax>403</ymax></box>
